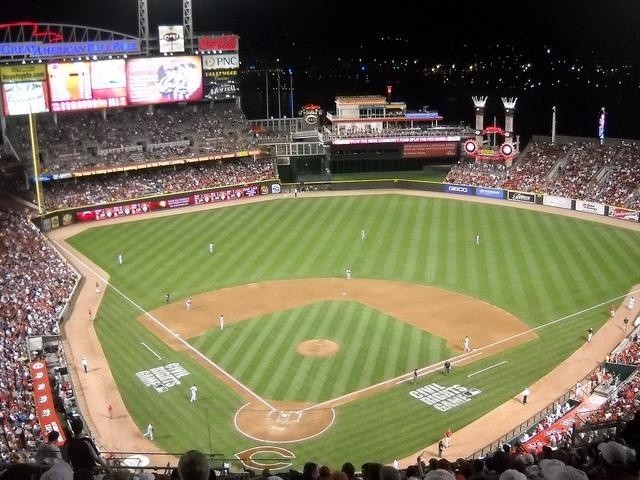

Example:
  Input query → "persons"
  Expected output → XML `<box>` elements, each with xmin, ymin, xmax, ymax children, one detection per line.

<box><xmin>510</xmin><ymin>327</ymin><xmax>640</xmax><ymax>479</ymax></box>
<box><xmin>443</xmin><ymin>361</ymin><xmax>452</xmax><ymax>376</ymax></box>
<box><xmin>412</xmin><ymin>368</ymin><xmax>418</xmax><ymax>384</ymax></box>
<box><xmin>360</xmin><ymin>227</ymin><xmax>368</xmax><ymax>239</ymax></box>
<box><xmin>442</xmin><ymin>135</ymin><xmax>640</xmax><ymax>210</ymax></box>
<box><xmin>0</xmin><ymin>101</ymin><xmax>333</xmax><ymax>213</ymax></box>
<box><xmin>185</xmin><ymin>296</ymin><xmax>192</xmax><ymax>311</ymax></box>
<box><xmin>141</xmin><ymin>421</ymin><xmax>156</xmax><ymax>440</ymax></box>
<box><xmin>622</xmin><ymin>316</ymin><xmax>629</xmax><ymax>332</ymax></box>
<box><xmin>219</xmin><ymin>314</ymin><xmax>225</xmax><ymax>331</ymax></box>
<box><xmin>0</xmin><ymin>213</ymin><xmax>113</xmax><ymax>479</ymax></box>
<box><xmin>609</xmin><ymin>304</ymin><xmax>615</xmax><ymax>317</ymax></box>
<box><xmin>153</xmin><ymin>61</ymin><xmax>201</xmax><ymax>102</ymax></box>
<box><xmin>462</xmin><ymin>335</ymin><xmax>472</xmax><ymax>351</ymax></box>
<box><xmin>442</xmin><ymin>429</ymin><xmax>454</xmax><ymax>448</ymax></box>
<box><xmin>333</xmin><ymin>109</ymin><xmax>478</xmax><ymax>141</ymax></box>
<box><xmin>117</xmin><ymin>254</ymin><xmax>123</xmax><ymax>264</ymax></box>
<box><xmin>627</xmin><ymin>296</ymin><xmax>635</xmax><ymax>310</ymax></box>
<box><xmin>113</xmin><ymin>440</ymin><xmax>509</xmax><ymax>480</ymax></box>
<box><xmin>190</xmin><ymin>384</ymin><xmax>197</xmax><ymax>402</ymax></box>
<box><xmin>344</xmin><ymin>266</ymin><xmax>352</xmax><ymax>279</ymax></box>
<box><xmin>473</xmin><ymin>233</ymin><xmax>481</xmax><ymax>247</ymax></box>
<box><xmin>208</xmin><ymin>241</ymin><xmax>216</xmax><ymax>255</ymax></box>
<box><xmin>164</xmin><ymin>292</ymin><xmax>169</xmax><ymax>304</ymax></box>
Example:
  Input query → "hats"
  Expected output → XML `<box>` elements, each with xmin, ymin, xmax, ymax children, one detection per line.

<box><xmin>597</xmin><ymin>441</ymin><xmax>636</xmax><ymax>464</ymax></box>
<box><xmin>424</xmin><ymin>469</ymin><xmax>457</xmax><ymax>480</ymax></box>
<box><xmin>499</xmin><ymin>454</ymin><xmax>589</xmax><ymax>480</ymax></box>
